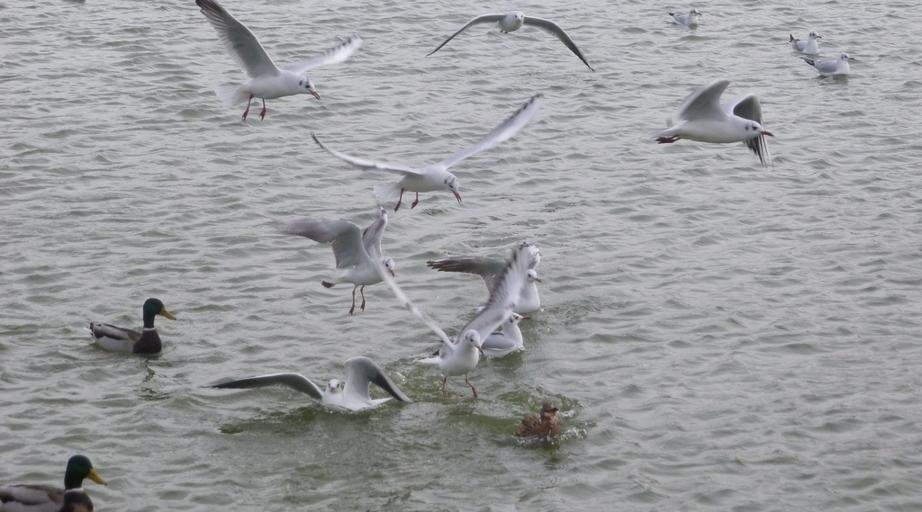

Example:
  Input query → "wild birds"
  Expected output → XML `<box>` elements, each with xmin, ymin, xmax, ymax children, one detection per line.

<box><xmin>653</xmin><ymin>79</ymin><xmax>774</xmax><ymax>168</ymax></box>
<box><xmin>663</xmin><ymin>7</ymin><xmax>702</xmax><ymax>26</ymax></box>
<box><xmin>371</xmin><ymin>240</ymin><xmax>548</xmax><ymax>402</ymax></box>
<box><xmin>269</xmin><ymin>206</ymin><xmax>396</xmax><ymax>316</ymax></box>
<box><xmin>511</xmin><ymin>405</ymin><xmax>561</xmax><ymax>437</ymax></box>
<box><xmin>425</xmin><ymin>10</ymin><xmax>596</xmax><ymax>72</ymax></box>
<box><xmin>789</xmin><ymin>30</ymin><xmax>855</xmax><ymax>76</ymax></box>
<box><xmin>305</xmin><ymin>92</ymin><xmax>547</xmax><ymax>214</ymax></box>
<box><xmin>195</xmin><ymin>0</ymin><xmax>364</xmax><ymax>121</ymax></box>
<box><xmin>197</xmin><ymin>355</ymin><xmax>414</xmax><ymax>413</ymax></box>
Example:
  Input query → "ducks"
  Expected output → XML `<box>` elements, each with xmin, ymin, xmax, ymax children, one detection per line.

<box><xmin>89</xmin><ymin>297</ymin><xmax>177</xmax><ymax>354</ymax></box>
<box><xmin>0</xmin><ymin>454</ymin><xmax>108</xmax><ymax>512</ymax></box>
